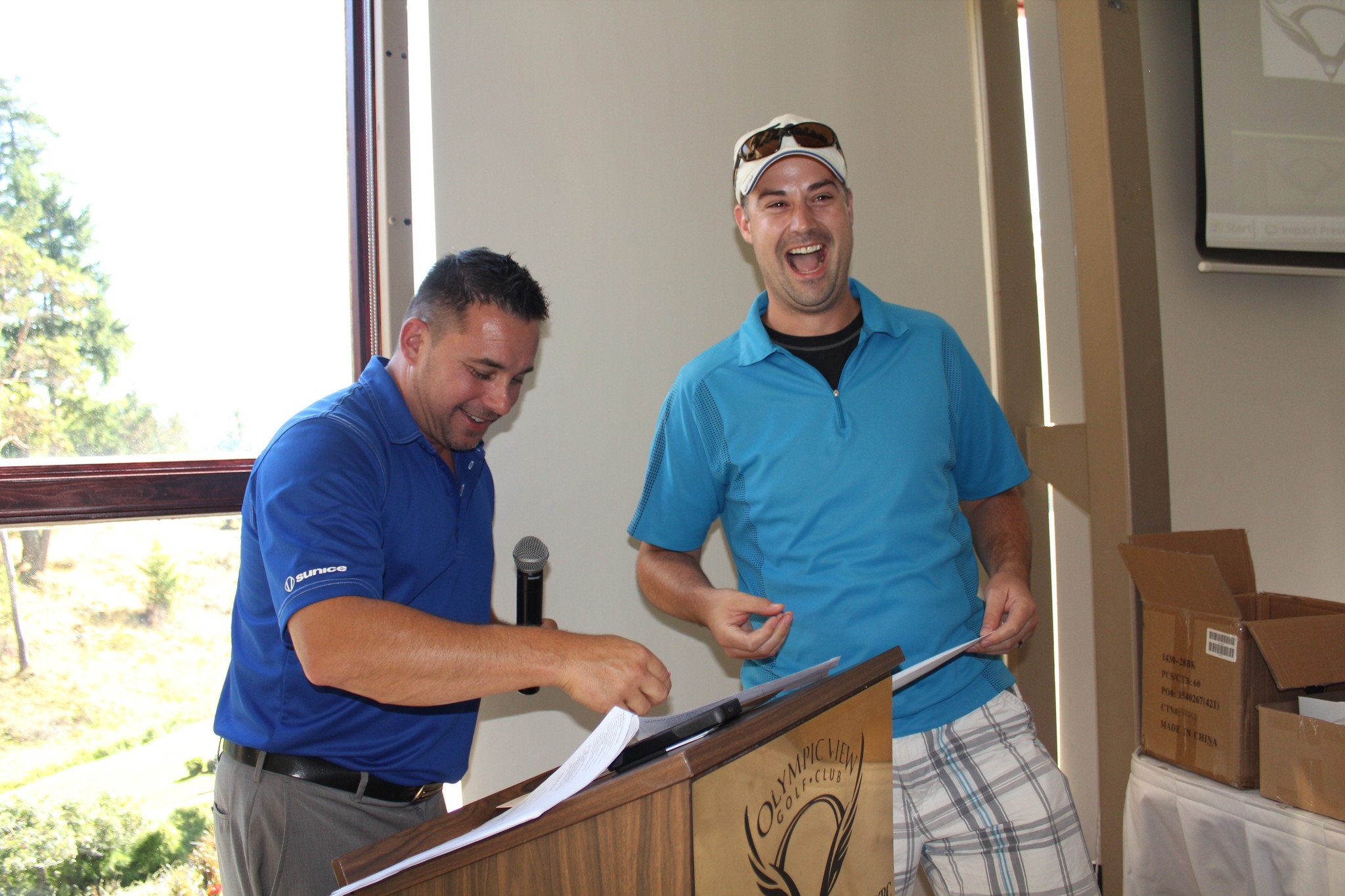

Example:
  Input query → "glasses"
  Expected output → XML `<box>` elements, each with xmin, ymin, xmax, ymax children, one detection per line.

<box><xmin>732</xmin><ymin>122</ymin><xmax>847</xmax><ymax>187</ymax></box>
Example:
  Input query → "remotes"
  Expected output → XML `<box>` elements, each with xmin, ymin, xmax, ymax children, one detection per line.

<box><xmin>608</xmin><ymin>699</ymin><xmax>743</xmax><ymax>772</ymax></box>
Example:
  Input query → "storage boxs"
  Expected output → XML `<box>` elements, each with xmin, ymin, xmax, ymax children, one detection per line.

<box><xmin>1118</xmin><ymin>529</ymin><xmax>1345</xmax><ymax>821</ymax></box>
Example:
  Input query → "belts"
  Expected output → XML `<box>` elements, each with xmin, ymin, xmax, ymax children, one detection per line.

<box><xmin>222</xmin><ymin>739</ymin><xmax>444</xmax><ymax>802</ymax></box>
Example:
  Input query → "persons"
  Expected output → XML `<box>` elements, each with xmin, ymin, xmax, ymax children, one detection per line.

<box><xmin>629</xmin><ymin>113</ymin><xmax>1101</xmax><ymax>896</ymax></box>
<box><xmin>212</xmin><ymin>247</ymin><xmax>671</xmax><ymax>896</ymax></box>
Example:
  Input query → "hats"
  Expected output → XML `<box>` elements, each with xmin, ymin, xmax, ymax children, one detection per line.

<box><xmin>732</xmin><ymin>114</ymin><xmax>846</xmax><ymax>205</ymax></box>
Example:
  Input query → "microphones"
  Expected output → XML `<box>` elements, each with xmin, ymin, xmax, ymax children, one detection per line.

<box><xmin>513</xmin><ymin>536</ymin><xmax>549</xmax><ymax>695</ymax></box>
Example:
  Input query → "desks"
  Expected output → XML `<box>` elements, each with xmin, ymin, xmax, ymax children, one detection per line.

<box><xmin>1122</xmin><ymin>747</ymin><xmax>1344</xmax><ymax>896</ymax></box>
